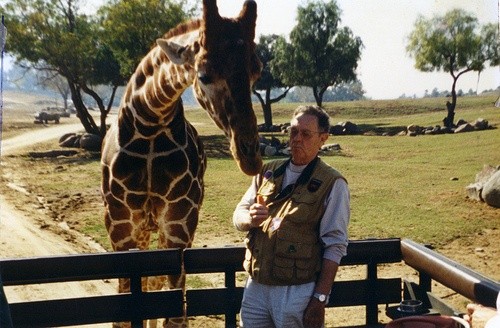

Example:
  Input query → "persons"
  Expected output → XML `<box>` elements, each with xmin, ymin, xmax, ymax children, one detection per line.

<box><xmin>463</xmin><ymin>290</ymin><xmax>500</xmax><ymax>328</ymax></box>
<box><xmin>232</xmin><ymin>104</ymin><xmax>351</xmax><ymax>328</ymax></box>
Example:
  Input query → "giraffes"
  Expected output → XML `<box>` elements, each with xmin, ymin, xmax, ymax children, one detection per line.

<box><xmin>101</xmin><ymin>0</ymin><xmax>266</xmax><ymax>328</ymax></box>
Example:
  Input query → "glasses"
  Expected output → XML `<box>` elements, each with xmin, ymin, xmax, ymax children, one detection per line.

<box><xmin>287</xmin><ymin>126</ymin><xmax>324</xmax><ymax>138</ymax></box>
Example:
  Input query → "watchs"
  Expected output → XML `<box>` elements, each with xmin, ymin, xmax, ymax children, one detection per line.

<box><xmin>312</xmin><ymin>292</ymin><xmax>328</xmax><ymax>303</ymax></box>
